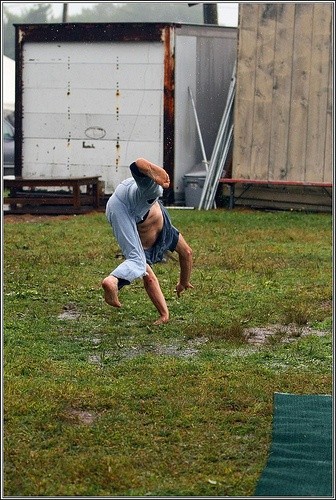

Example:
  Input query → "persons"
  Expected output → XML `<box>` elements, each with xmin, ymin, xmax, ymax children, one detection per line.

<box><xmin>100</xmin><ymin>158</ymin><xmax>194</xmax><ymax>326</ymax></box>
